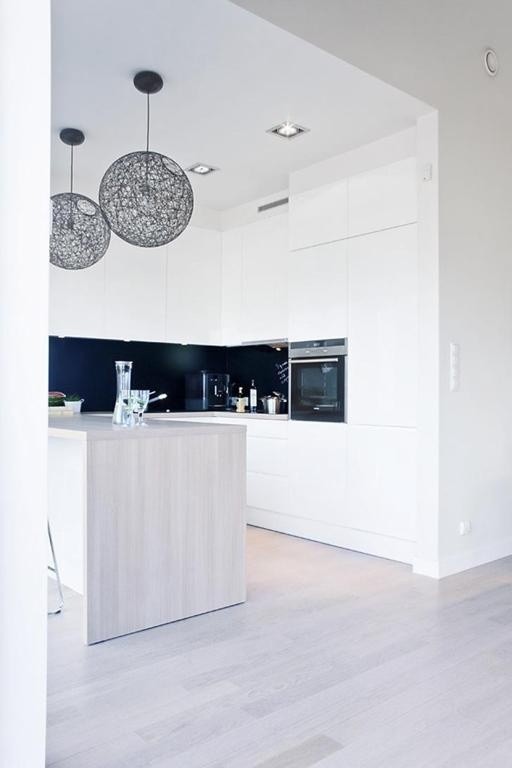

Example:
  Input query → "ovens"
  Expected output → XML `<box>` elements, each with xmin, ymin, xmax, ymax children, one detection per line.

<box><xmin>288</xmin><ymin>337</ymin><xmax>347</xmax><ymax>424</ymax></box>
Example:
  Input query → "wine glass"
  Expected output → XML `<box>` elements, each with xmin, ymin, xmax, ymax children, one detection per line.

<box><xmin>119</xmin><ymin>388</ymin><xmax>150</xmax><ymax>431</ymax></box>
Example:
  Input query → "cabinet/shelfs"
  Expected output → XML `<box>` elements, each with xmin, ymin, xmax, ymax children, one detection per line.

<box><xmin>164</xmin><ymin>231</ymin><xmax>222</xmax><ymax>346</ymax></box>
<box><xmin>345</xmin><ymin>156</ymin><xmax>417</xmax><ymax>424</ymax></box>
<box><xmin>222</xmin><ymin>213</ymin><xmax>286</xmax><ymax>345</ymax></box>
<box><xmin>248</xmin><ymin>427</ymin><xmax>286</xmax><ymax>535</ymax></box>
<box><xmin>286</xmin><ymin>432</ymin><xmax>414</xmax><ymax>565</ymax></box>
<box><xmin>288</xmin><ymin>179</ymin><xmax>349</xmax><ymax>341</ymax></box>
<box><xmin>50</xmin><ymin>230</ymin><xmax>164</xmax><ymax>344</ymax></box>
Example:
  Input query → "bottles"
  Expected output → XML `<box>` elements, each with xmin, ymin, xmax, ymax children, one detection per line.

<box><xmin>235</xmin><ymin>378</ymin><xmax>259</xmax><ymax>413</ymax></box>
<box><xmin>112</xmin><ymin>360</ymin><xmax>134</xmax><ymax>425</ymax></box>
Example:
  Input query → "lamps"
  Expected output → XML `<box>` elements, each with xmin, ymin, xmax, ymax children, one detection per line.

<box><xmin>99</xmin><ymin>71</ymin><xmax>194</xmax><ymax>249</ymax></box>
<box><xmin>50</xmin><ymin>127</ymin><xmax>110</xmax><ymax>271</ymax></box>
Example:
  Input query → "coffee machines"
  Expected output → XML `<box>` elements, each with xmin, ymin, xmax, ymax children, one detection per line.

<box><xmin>183</xmin><ymin>371</ymin><xmax>231</xmax><ymax>411</ymax></box>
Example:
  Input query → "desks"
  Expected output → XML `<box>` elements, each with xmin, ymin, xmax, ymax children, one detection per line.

<box><xmin>48</xmin><ymin>416</ymin><xmax>247</xmax><ymax>646</ymax></box>
